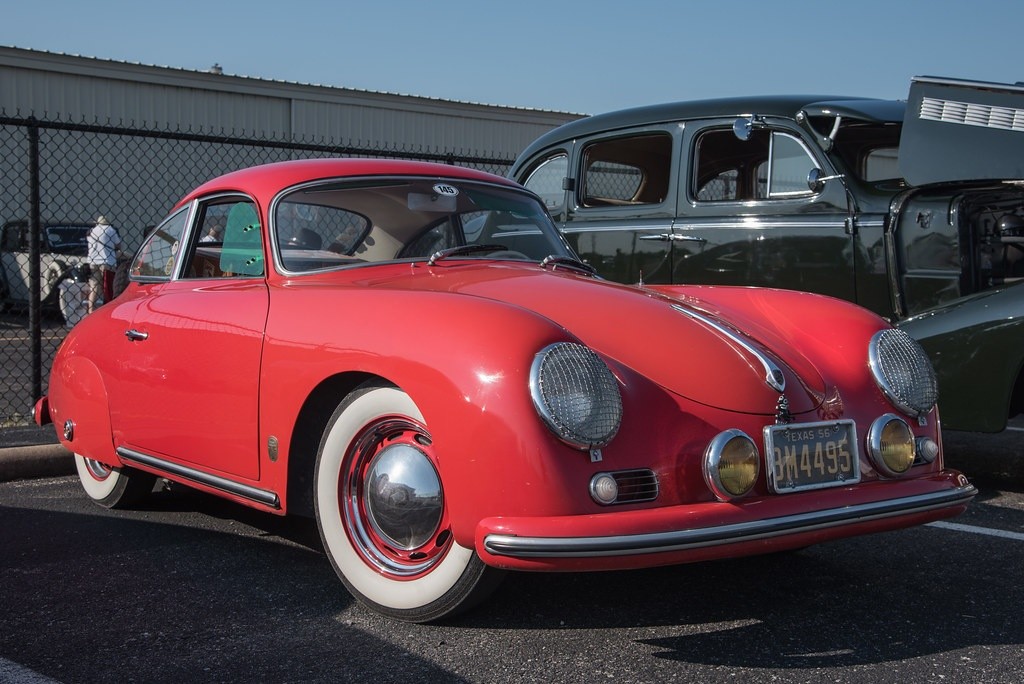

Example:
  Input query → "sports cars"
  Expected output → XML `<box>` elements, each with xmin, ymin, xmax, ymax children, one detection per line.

<box><xmin>33</xmin><ymin>159</ymin><xmax>982</xmax><ymax>624</ymax></box>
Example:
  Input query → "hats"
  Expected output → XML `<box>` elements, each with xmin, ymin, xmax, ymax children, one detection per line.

<box><xmin>93</xmin><ymin>215</ymin><xmax>110</xmax><ymax>225</ymax></box>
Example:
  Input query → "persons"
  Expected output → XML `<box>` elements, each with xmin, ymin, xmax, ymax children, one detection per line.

<box><xmin>88</xmin><ymin>215</ymin><xmax>120</xmax><ymax>315</ymax></box>
<box><xmin>201</xmin><ymin>225</ymin><xmax>223</xmax><ymax>242</ymax></box>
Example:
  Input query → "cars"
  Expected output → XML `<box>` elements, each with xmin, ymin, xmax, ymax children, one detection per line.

<box><xmin>505</xmin><ymin>94</ymin><xmax>1024</xmax><ymax>317</ymax></box>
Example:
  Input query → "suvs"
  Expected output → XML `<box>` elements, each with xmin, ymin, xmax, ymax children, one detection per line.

<box><xmin>0</xmin><ymin>220</ymin><xmax>120</xmax><ymax>322</ymax></box>
